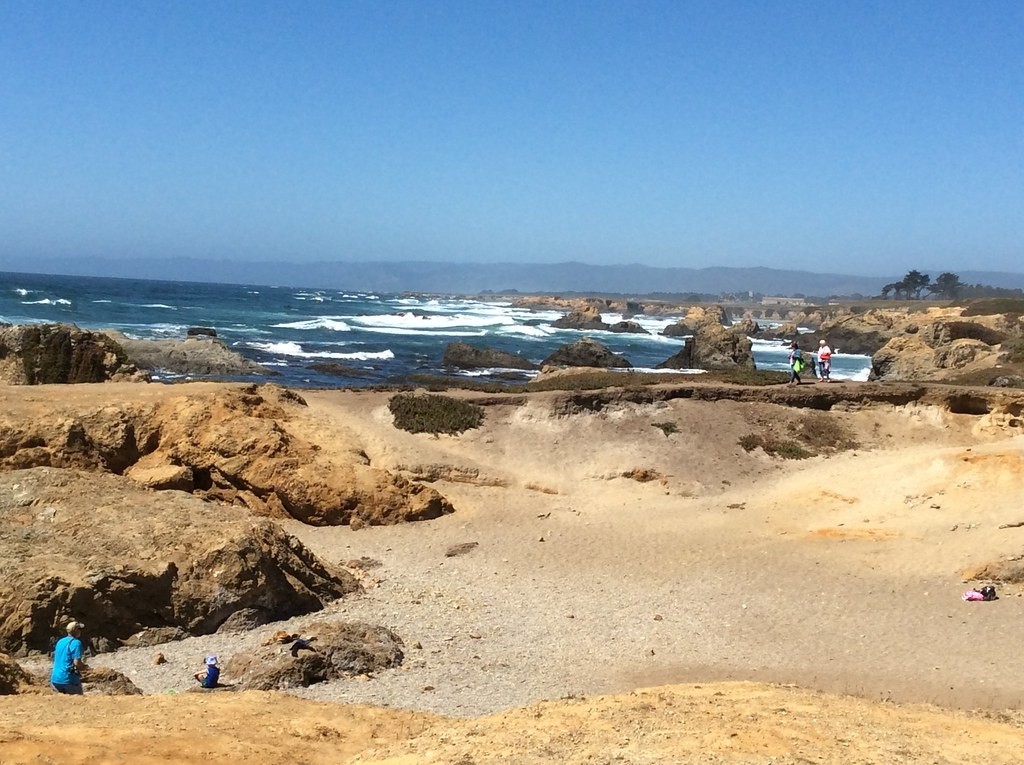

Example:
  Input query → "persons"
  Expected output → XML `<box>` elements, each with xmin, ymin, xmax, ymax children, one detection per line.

<box><xmin>193</xmin><ymin>656</ymin><xmax>220</xmax><ymax>688</ymax></box>
<box><xmin>51</xmin><ymin>621</ymin><xmax>88</xmax><ymax>695</ymax></box>
<box><xmin>818</xmin><ymin>339</ymin><xmax>832</xmax><ymax>383</ymax></box>
<box><xmin>786</xmin><ymin>343</ymin><xmax>802</xmax><ymax>387</ymax></box>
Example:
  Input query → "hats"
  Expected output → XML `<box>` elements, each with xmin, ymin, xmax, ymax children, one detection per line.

<box><xmin>66</xmin><ymin>621</ymin><xmax>84</xmax><ymax>632</ymax></box>
<box><xmin>206</xmin><ymin>656</ymin><xmax>218</xmax><ymax>665</ymax></box>
